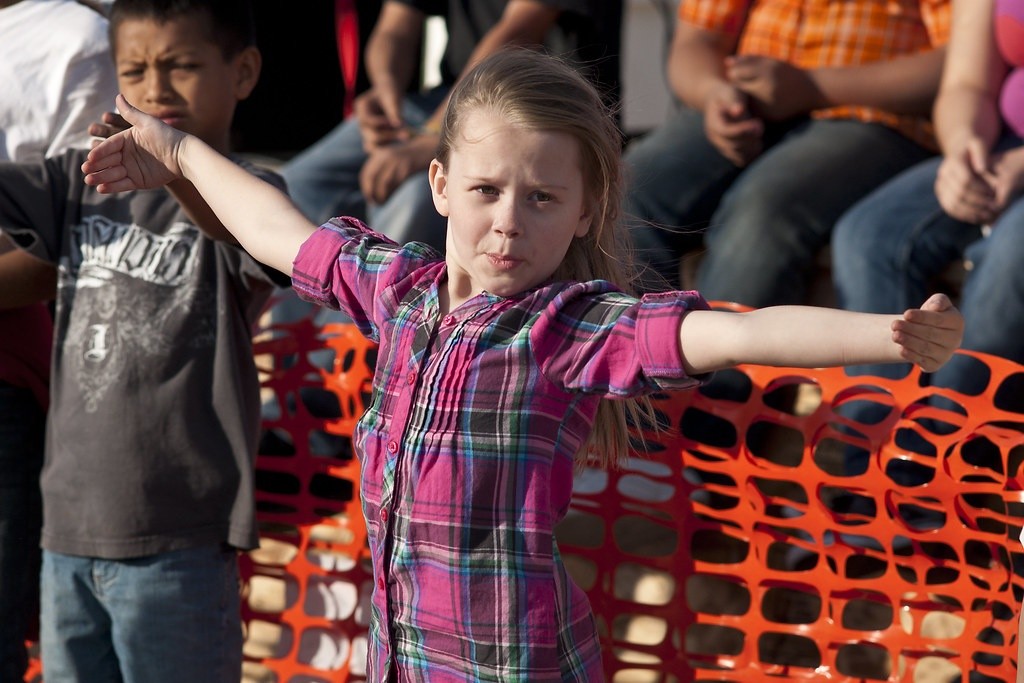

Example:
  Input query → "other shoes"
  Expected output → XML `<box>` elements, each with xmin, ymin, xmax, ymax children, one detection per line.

<box><xmin>260</xmin><ymin>399</ymin><xmax>334</xmax><ymax>493</ymax></box>
<box><xmin>784</xmin><ymin>503</ymin><xmax>946</xmax><ymax>573</ymax></box>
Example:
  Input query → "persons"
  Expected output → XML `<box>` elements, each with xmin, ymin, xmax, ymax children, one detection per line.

<box><xmin>614</xmin><ymin>0</ymin><xmax>956</xmax><ymax>529</ymax></box>
<box><xmin>0</xmin><ymin>0</ymin><xmax>289</xmax><ymax>683</ymax></box>
<box><xmin>82</xmin><ymin>52</ymin><xmax>964</xmax><ymax>683</ymax></box>
<box><xmin>785</xmin><ymin>1</ymin><xmax>1024</xmax><ymax>584</ymax></box>
<box><xmin>257</xmin><ymin>0</ymin><xmax>600</xmax><ymax>487</ymax></box>
